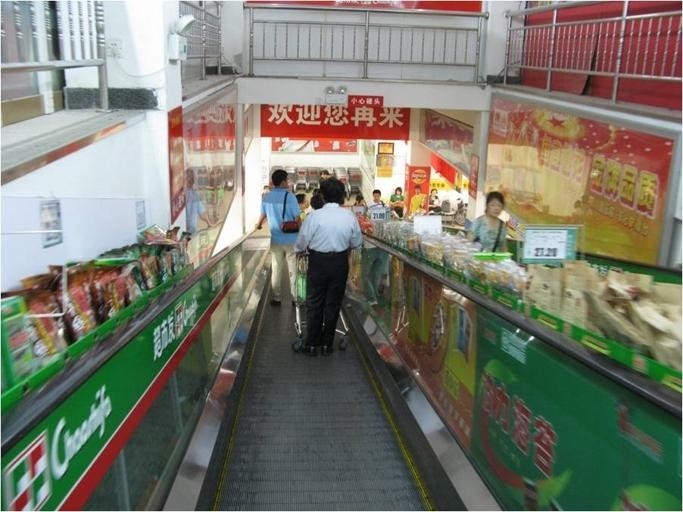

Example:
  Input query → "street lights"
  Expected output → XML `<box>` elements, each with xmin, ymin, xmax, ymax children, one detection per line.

<box><xmin>281</xmin><ymin>221</ymin><xmax>299</xmax><ymax>233</ymax></box>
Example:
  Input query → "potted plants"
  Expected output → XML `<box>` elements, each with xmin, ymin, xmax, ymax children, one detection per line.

<box><xmin>377</xmin><ymin>142</ymin><xmax>395</xmax><ymax>155</ymax></box>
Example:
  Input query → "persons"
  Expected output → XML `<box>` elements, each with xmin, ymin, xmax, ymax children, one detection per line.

<box><xmin>389</xmin><ymin>186</ymin><xmax>404</xmax><ymax>218</ymax></box>
<box><xmin>366</xmin><ymin>189</ymin><xmax>386</xmax><ymax>209</ymax></box>
<box><xmin>295</xmin><ymin>193</ymin><xmax>310</xmax><ymax>220</ymax></box>
<box><xmin>429</xmin><ymin>188</ymin><xmax>440</xmax><ymax>212</ymax></box>
<box><xmin>320</xmin><ymin>169</ymin><xmax>332</xmax><ymax>180</ymax></box>
<box><xmin>466</xmin><ymin>191</ymin><xmax>508</xmax><ymax>252</ymax></box>
<box><xmin>258</xmin><ymin>170</ymin><xmax>302</xmax><ymax>306</ymax></box>
<box><xmin>185</xmin><ymin>168</ymin><xmax>215</xmax><ymax>239</ymax></box>
<box><xmin>361</xmin><ymin>239</ymin><xmax>388</xmax><ymax>305</ymax></box>
<box><xmin>351</xmin><ymin>194</ymin><xmax>365</xmax><ymax>217</ymax></box>
<box><xmin>294</xmin><ymin>177</ymin><xmax>363</xmax><ymax>357</ymax></box>
<box><xmin>409</xmin><ymin>186</ymin><xmax>424</xmax><ymax>216</ymax></box>
<box><xmin>310</xmin><ymin>196</ymin><xmax>324</xmax><ymax>211</ymax></box>
<box><xmin>262</xmin><ymin>186</ymin><xmax>270</xmax><ymax>197</ymax></box>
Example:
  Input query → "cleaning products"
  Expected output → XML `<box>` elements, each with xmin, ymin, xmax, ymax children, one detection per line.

<box><xmin>195</xmin><ymin>227</ymin><xmax>216</xmax><ymax>249</ymax></box>
<box><xmin>289</xmin><ymin>250</ymin><xmax>352</xmax><ymax>355</ymax></box>
<box><xmin>267</xmin><ymin>163</ymin><xmax>364</xmax><ymax>201</ymax></box>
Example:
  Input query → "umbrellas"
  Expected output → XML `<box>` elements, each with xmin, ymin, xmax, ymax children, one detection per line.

<box><xmin>302</xmin><ymin>345</ymin><xmax>317</xmax><ymax>356</ymax></box>
<box><xmin>322</xmin><ymin>346</ymin><xmax>333</xmax><ymax>356</ymax></box>
